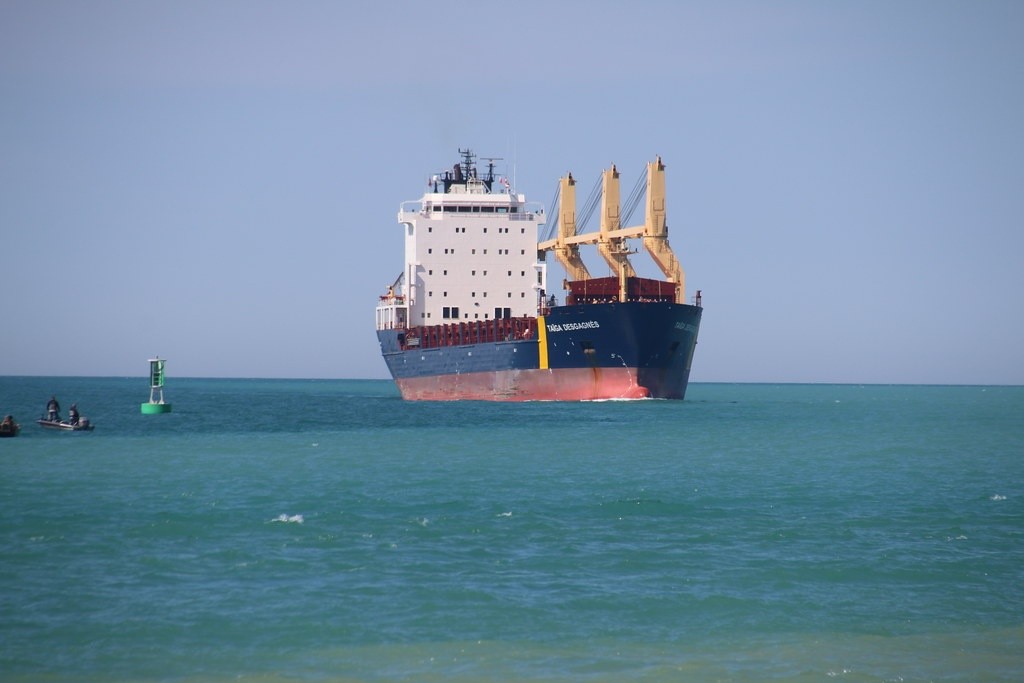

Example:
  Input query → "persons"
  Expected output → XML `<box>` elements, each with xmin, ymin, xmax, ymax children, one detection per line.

<box><xmin>592</xmin><ymin>296</ymin><xmax>604</xmax><ymax>304</ymax></box>
<box><xmin>550</xmin><ymin>293</ymin><xmax>556</xmax><ymax>301</ymax></box>
<box><xmin>47</xmin><ymin>397</ymin><xmax>60</xmax><ymax>422</ymax></box>
<box><xmin>69</xmin><ymin>403</ymin><xmax>79</xmax><ymax>425</ymax></box>
<box><xmin>1</xmin><ymin>414</ymin><xmax>17</xmax><ymax>429</ymax></box>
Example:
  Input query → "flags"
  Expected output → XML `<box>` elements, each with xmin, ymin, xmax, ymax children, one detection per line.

<box><xmin>504</xmin><ymin>179</ymin><xmax>511</xmax><ymax>192</ymax></box>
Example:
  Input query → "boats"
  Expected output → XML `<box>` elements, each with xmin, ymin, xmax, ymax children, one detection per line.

<box><xmin>0</xmin><ymin>423</ymin><xmax>20</xmax><ymax>438</ymax></box>
<box><xmin>140</xmin><ymin>355</ymin><xmax>173</xmax><ymax>415</ymax></box>
<box><xmin>374</xmin><ymin>147</ymin><xmax>706</xmax><ymax>402</ymax></box>
<box><xmin>35</xmin><ymin>418</ymin><xmax>95</xmax><ymax>432</ymax></box>
